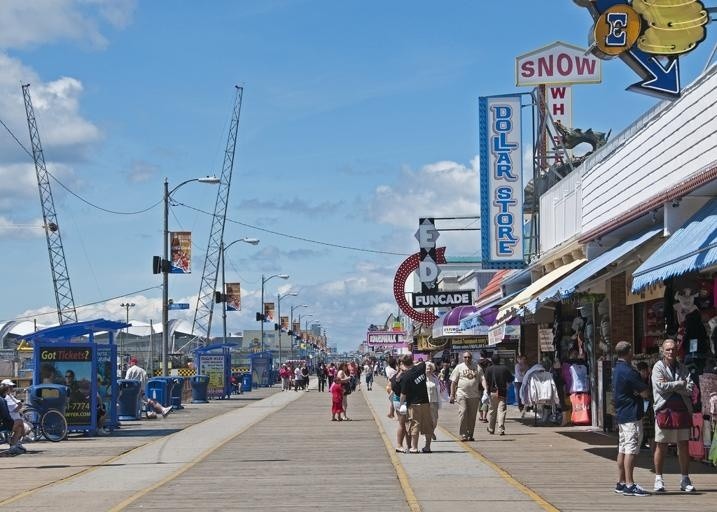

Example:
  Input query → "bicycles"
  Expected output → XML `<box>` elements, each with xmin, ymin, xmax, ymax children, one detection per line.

<box><xmin>0</xmin><ymin>394</ymin><xmax>67</xmax><ymax>445</ymax></box>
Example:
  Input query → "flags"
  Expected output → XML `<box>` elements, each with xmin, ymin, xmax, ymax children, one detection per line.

<box><xmin>225</xmin><ymin>282</ymin><xmax>243</xmax><ymax>313</ymax></box>
<box><xmin>263</xmin><ymin>300</ymin><xmax>275</xmax><ymax>323</ymax></box>
<box><xmin>168</xmin><ymin>230</ymin><xmax>192</xmax><ymax>276</ymax></box>
<box><xmin>280</xmin><ymin>316</ymin><xmax>327</xmax><ymax>352</ymax></box>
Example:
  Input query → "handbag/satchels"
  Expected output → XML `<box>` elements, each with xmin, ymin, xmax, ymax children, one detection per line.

<box><xmin>341</xmin><ymin>381</ymin><xmax>351</xmax><ymax>395</ymax></box>
<box><xmin>656</xmin><ymin>399</ymin><xmax>692</xmax><ymax>430</ymax></box>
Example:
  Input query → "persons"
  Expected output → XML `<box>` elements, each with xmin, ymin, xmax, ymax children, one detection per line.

<box><xmin>139</xmin><ymin>389</ymin><xmax>173</xmax><ymax>419</ymax></box>
<box><xmin>230</xmin><ymin>371</ymin><xmax>238</xmax><ymax>389</ymax></box>
<box><xmin>93</xmin><ymin>392</ymin><xmax>112</xmax><ymax>436</ymax></box>
<box><xmin>62</xmin><ymin>369</ymin><xmax>82</xmax><ymax>399</ymax></box>
<box><xmin>96</xmin><ymin>360</ymin><xmax>112</xmax><ymax>401</ymax></box>
<box><xmin>236</xmin><ymin>371</ymin><xmax>245</xmax><ymax>395</ymax></box>
<box><xmin>1</xmin><ymin>378</ymin><xmax>34</xmax><ymax>453</ymax></box>
<box><xmin>277</xmin><ymin>347</ymin><xmax>530</xmax><ymax>454</ymax></box>
<box><xmin>648</xmin><ymin>337</ymin><xmax>698</xmax><ymax>495</ymax></box>
<box><xmin>0</xmin><ymin>382</ymin><xmax>24</xmax><ymax>455</ymax></box>
<box><xmin>35</xmin><ymin>363</ymin><xmax>59</xmax><ymax>397</ymax></box>
<box><xmin>124</xmin><ymin>357</ymin><xmax>149</xmax><ymax>401</ymax></box>
<box><xmin>634</xmin><ymin>361</ymin><xmax>653</xmax><ymax>448</ymax></box>
<box><xmin>608</xmin><ymin>341</ymin><xmax>652</xmax><ymax>497</ymax></box>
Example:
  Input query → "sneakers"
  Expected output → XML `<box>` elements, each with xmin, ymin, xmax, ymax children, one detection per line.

<box><xmin>653</xmin><ymin>479</ymin><xmax>665</xmax><ymax>491</ymax></box>
<box><xmin>487</xmin><ymin>427</ymin><xmax>494</xmax><ymax>434</ymax></box>
<box><xmin>396</xmin><ymin>446</ymin><xmax>430</xmax><ymax>453</ymax></box>
<box><xmin>462</xmin><ymin>434</ymin><xmax>474</xmax><ymax>441</ymax></box>
<box><xmin>500</xmin><ymin>431</ymin><xmax>504</xmax><ymax>435</ymax></box>
<box><xmin>680</xmin><ymin>476</ymin><xmax>696</xmax><ymax>492</ymax></box>
<box><xmin>161</xmin><ymin>405</ymin><xmax>173</xmax><ymax>416</ymax></box>
<box><xmin>615</xmin><ymin>482</ymin><xmax>652</xmax><ymax>496</ymax></box>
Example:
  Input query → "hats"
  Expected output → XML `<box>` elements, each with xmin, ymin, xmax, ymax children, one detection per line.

<box><xmin>129</xmin><ymin>357</ymin><xmax>136</xmax><ymax>364</ymax></box>
<box><xmin>1</xmin><ymin>379</ymin><xmax>16</xmax><ymax>386</ymax></box>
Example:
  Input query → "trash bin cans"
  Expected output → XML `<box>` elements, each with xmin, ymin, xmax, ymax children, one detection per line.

<box><xmin>241</xmin><ymin>373</ymin><xmax>252</xmax><ymax>392</ymax></box>
<box><xmin>116</xmin><ymin>379</ymin><xmax>141</xmax><ymax>421</ymax></box>
<box><xmin>145</xmin><ymin>376</ymin><xmax>185</xmax><ymax>412</ymax></box>
<box><xmin>191</xmin><ymin>375</ymin><xmax>210</xmax><ymax>403</ymax></box>
<box><xmin>27</xmin><ymin>384</ymin><xmax>72</xmax><ymax>441</ymax></box>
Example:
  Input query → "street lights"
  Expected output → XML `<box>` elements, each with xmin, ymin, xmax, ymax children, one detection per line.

<box><xmin>276</xmin><ymin>292</ymin><xmax>299</xmax><ymax>368</ymax></box>
<box><xmin>260</xmin><ymin>271</ymin><xmax>289</xmax><ymax>352</ymax></box>
<box><xmin>161</xmin><ymin>172</ymin><xmax>221</xmax><ymax>375</ymax></box>
<box><xmin>120</xmin><ymin>302</ymin><xmax>136</xmax><ymax>354</ymax></box>
<box><xmin>291</xmin><ymin>304</ymin><xmax>328</xmax><ymax>369</ymax></box>
<box><xmin>221</xmin><ymin>237</ymin><xmax>260</xmax><ymax>346</ymax></box>
